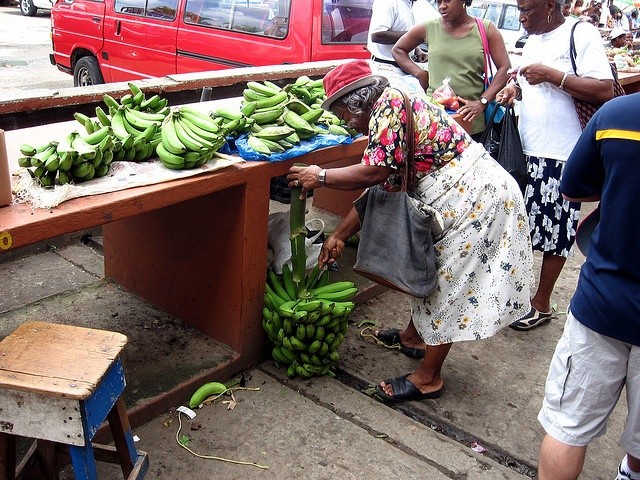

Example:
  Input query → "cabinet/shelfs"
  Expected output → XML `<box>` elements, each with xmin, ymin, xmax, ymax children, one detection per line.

<box><xmin>0</xmin><ymin>56</ymin><xmax>391</xmax><ymax>453</ymax></box>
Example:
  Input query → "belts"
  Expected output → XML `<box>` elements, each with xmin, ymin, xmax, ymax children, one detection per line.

<box><xmin>374</xmin><ymin>54</ymin><xmax>397</xmax><ymax>66</ymax></box>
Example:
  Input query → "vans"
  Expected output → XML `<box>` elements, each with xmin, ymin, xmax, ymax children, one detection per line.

<box><xmin>464</xmin><ymin>0</ymin><xmax>528</xmax><ymax>44</ymax></box>
<box><xmin>48</xmin><ymin>0</ymin><xmax>374</xmax><ymax>88</ymax></box>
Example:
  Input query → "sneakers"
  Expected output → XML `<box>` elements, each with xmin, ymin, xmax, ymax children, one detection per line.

<box><xmin>614</xmin><ymin>452</ymin><xmax>637</xmax><ymax>479</ymax></box>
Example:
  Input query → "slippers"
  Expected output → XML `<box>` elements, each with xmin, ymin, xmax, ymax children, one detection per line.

<box><xmin>373</xmin><ymin>325</ymin><xmax>426</xmax><ymax>360</ymax></box>
<box><xmin>509</xmin><ymin>305</ymin><xmax>552</xmax><ymax>331</ymax></box>
<box><xmin>375</xmin><ymin>373</ymin><xmax>446</xmax><ymax>402</ymax></box>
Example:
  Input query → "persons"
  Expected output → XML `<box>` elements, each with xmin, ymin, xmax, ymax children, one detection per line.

<box><xmin>628</xmin><ymin>10</ymin><xmax>640</xmax><ymax>34</ymax></box>
<box><xmin>564</xmin><ymin>0</ymin><xmax>574</xmax><ymax>16</ymax></box>
<box><xmin>533</xmin><ymin>88</ymin><xmax>639</xmax><ymax>480</ymax></box>
<box><xmin>493</xmin><ymin>1</ymin><xmax>614</xmax><ymax>333</ymax></box>
<box><xmin>285</xmin><ymin>58</ymin><xmax>534</xmax><ymax>405</ymax></box>
<box><xmin>625</xmin><ymin>32</ymin><xmax>634</xmax><ymax>44</ymax></box>
<box><xmin>603</xmin><ymin>26</ymin><xmax>627</xmax><ymax>50</ymax></box>
<box><xmin>580</xmin><ymin>2</ymin><xmax>602</xmax><ymax>21</ymax></box>
<box><xmin>365</xmin><ymin>0</ymin><xmax>441</xmax><ymax>65</ymax></box>
<box><xmin>605</xmin><ymin>5</ymin><xmax>629</xmax><ymax>30</ymax></box>
<box><xmin>587</xmin><ymin>14</ymin><xmax>599</xmax><ymax>26</ymax></box>
<box><xmin>390</xmin><ymin>1</ymin><xmax>511</xmax><ymax>145</ymax></box>
<box><xmin>571</xmin><ymin>0</ymin><xmax>584</xmax><ymax>16</ymax></box>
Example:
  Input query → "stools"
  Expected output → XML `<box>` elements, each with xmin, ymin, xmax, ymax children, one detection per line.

<box><xmin>0</xmin><ymin>320</ymin><xmax>151</xmax><ymax>480</ymax></box>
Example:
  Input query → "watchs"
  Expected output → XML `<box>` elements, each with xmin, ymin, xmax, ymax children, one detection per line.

<box><xmin>318</xmin><ymin>168</ymin><xmax>327</xmax><ymax>187</ymax></box>
<box><xmin>557</xmin><ymin>73</ymin><xmax>569</xmax><ymax>92</ymax></box>
<box><xmin>479</xmin><ymin>96</ymin><xmax>489</xmax><ymax>107</ymax></box>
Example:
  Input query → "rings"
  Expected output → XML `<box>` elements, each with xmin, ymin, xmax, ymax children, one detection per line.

<box><xmin>294</xmin><ymin>180</ymin><xmax>299</xmax><ymax>187</ymax></box>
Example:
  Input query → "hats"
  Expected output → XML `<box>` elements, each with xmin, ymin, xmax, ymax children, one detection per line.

<box><xmin>610</xmin><ymin>27</ymin><xmax>629</xmax><ymax>40</ymax></box>
<box><xmin>318</xmin><ymin>60</ymin><xmax>390</xmax><ymax>109</ymax></box>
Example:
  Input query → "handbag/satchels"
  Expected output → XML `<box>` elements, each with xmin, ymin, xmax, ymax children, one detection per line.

<box><xmin>351</xmin><ymin>185</ymin><xmax>446</xmax><ymax>299</ymax></box>
<box><xmin>566</xmin><ymin>20</ymin><xmax>629</xmax><ymax>132</ymax></box>
<box><xmin>484</xmin><ymin>73</ymin><xmax>505</xmax><ymax>128</ymax></box>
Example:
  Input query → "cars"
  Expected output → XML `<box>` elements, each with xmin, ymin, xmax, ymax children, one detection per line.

<box><xmin>15</xmin><ymin>0</ymin><xmax>58</xmax><ymax>16</ymax></box>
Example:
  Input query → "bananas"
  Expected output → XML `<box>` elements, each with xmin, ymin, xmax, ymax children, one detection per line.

<box><xmin>156</xmin><ymin>108</ymin><xmax>227</xmax><ymax>170</ymax></box>
<box><xmin>74</xmin><ymin>82</ymin><xmax>170</xmax><ymax>161</ymax></box>
<box><xmin>263</xmin><ymin>263</ymin><xmax>358</xmax><ymax>378</ymax></box>
<box><xmin>240</xmin><ymin>76</ymin><xmax>358</xmax><ymax>152</ymax></box>
<box><xmin>189</xmin><ymin>382</ymin><xmax>230</xmax><ymax>410</ymax></box>
<box><xmin>209</xmin><ymin>111</ymin><xmax>255</xmax><ymax>137</ymax></box>
<box><xmin>19</xmin><ymin>126</ymin><xmax>114</xmax><ymax>186</ymax></box>
